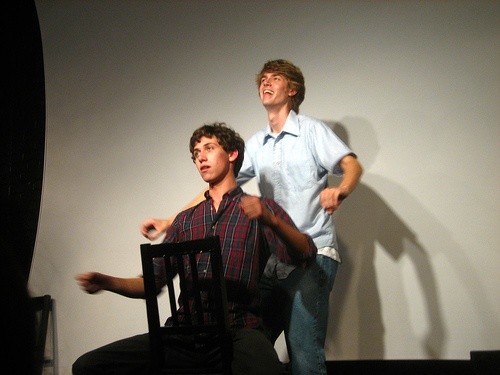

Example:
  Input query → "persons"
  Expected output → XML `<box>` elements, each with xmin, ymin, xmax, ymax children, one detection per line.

<box><xmin>139</xmin><ymin>58</ymin><xmax>363</xmax><ymax>375</ymax></box>
<box><xmin>71</xmin><ymin>121</ymin><xmax>318</xmax><ymax>375</ymax></box>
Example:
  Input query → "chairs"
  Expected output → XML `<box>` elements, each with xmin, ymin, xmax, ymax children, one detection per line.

<box><xmin>32</xmin><ymin>294</ymin><xmax>51</xmax><ymax>375</ymax></box>
<box><xmin>141</xmin><ymin>236</ymin><xmax>286</xmax><ymax>375</ymax></box>
